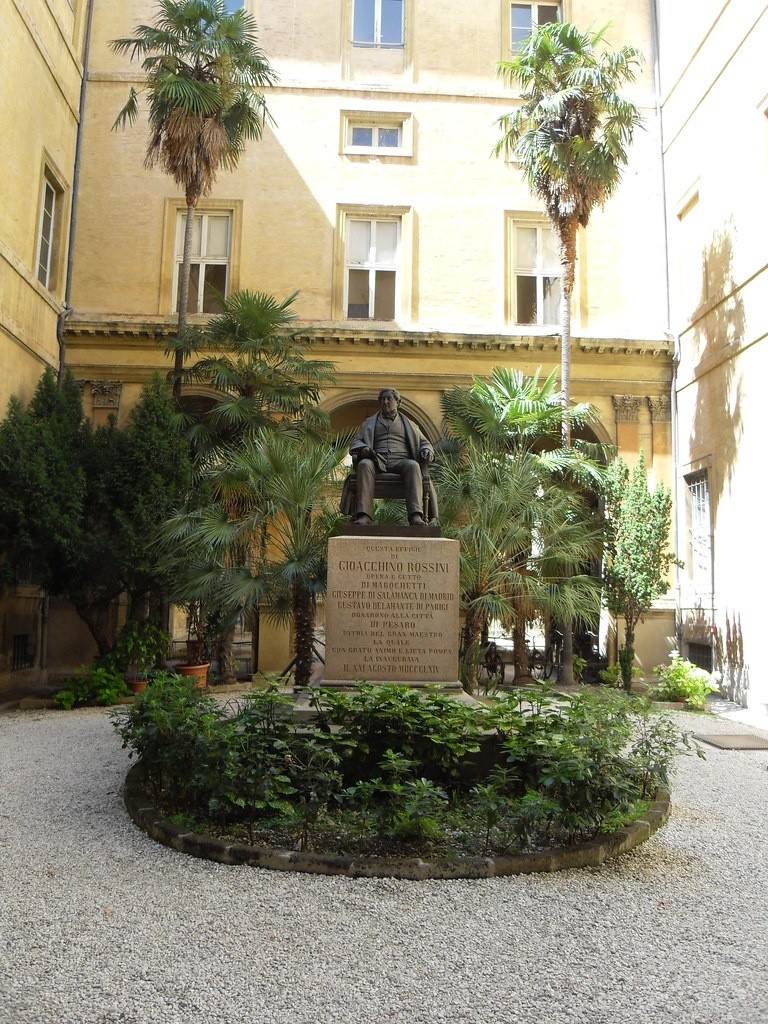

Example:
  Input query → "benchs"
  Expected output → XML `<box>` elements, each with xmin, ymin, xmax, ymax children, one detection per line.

<box><xmin>171</xmin><ymin>656</ymin><xmax>253</xmax><ymax>674</ymax></box>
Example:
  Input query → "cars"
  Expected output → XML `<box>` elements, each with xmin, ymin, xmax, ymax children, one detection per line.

<box><xmin>545</xmin><ymin>630</ymin><xmax>599</xmax><ymax>658</ymax></box>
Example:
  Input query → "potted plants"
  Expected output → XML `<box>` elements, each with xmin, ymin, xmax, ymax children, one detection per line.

<box><xmin>114</xmin><ymin>617</ymin><xmax>172</xmax><ymax>693</ymax></box>
<box><xmin>174</xmin><ymin>601</ymin><xmax>210</xmax><ymax>689</ymax></box>
<box><xmin>650</xmin><ymin>654</ymin><xmax>721</xmax><ymax>711</ymax></box>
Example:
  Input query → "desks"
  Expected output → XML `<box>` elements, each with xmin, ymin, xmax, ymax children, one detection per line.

<box><xmin>173</xmin><ymin>640</ymin><xmax>252</xmax><ymax>646</ymax></box>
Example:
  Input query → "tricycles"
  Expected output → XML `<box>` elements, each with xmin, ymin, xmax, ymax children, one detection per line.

<box><xmin>478</xmin><ymin>633</ymin><xmax>506</xmax><ymax>684</ymax></box>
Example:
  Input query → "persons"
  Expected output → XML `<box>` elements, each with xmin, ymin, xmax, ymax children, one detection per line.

<box><xmin>349</xmin><ymin>388</ymin><xmax>435</xmax><ymax>526</ymax></box>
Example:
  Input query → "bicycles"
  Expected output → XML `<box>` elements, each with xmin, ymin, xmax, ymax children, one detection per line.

<box><xmin>525</xmin><ymin>634</ymin><xmax>544</xmax><ymax>679</ymax></box>
<box><xmin>545</xmin><ymin>628</ymin><xmax>581</xmax><ymax>682</ymax></box>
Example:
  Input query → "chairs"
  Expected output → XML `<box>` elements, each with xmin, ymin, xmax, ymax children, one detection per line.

<box><xmin>348</xmin><ymin>452</ymin><xmax>435</xmax><ymax>524</ymax></box>
